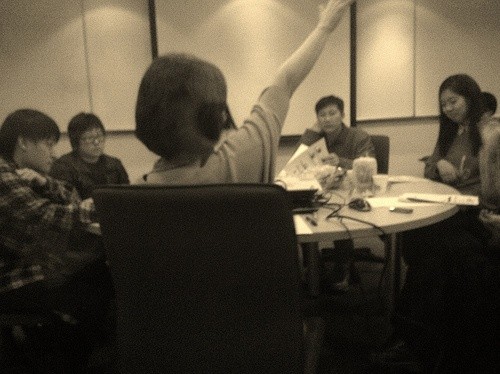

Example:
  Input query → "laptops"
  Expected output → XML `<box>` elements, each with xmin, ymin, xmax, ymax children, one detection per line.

<box><xmin>288</xmin><ymin>189</ymin><xmax>320</xmax><ymax>214</ymax></box>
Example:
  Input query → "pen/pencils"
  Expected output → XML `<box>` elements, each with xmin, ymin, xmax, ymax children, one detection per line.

<box><xmin>306</xmin><ymin>216</ymin><xmax>318</xmax><ymax>226</ymax></box>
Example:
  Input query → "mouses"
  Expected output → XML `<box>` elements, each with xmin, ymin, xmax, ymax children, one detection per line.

<box><xmin>349</xmin><ymin>198</ymin><xmax>372</xmax><ymax>212</ymax></box>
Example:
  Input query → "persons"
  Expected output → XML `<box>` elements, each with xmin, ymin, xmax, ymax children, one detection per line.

<box><xmin>48</xmin><ymin>112</ymin><xmax>129</xmax><ymax>199</ymax></box>
<box><xmin>368</xmin><ymin>75</ymin><xmax>500</xmax><ymax>374</ymax></box>
<box><xmin>300</xmin><ymin>95</ymin><xmax>376</xmax><ymax>291</ymax></box>
<box><xmin>1</xmin><ymin>108</ymin><xmax>107</xmax><ymax>314</ymax></box>
<box><xmin>130</xmin><ymin>0</ymin><xmax>353</xmax><ymax>186</ymax></box>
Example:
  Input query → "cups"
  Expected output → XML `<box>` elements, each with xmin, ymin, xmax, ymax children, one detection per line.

<box><xmin>354</xmin><ymin>156</ymin><xmax>378</xmax><ymax>198</ymax></box>
<box><xmin>372</xmin><ymin>174</ymin><xmax>389</xmax><ymax>196</ymax></box>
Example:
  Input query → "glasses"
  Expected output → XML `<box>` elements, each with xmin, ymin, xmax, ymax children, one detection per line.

<box><xmin>80</xmin><ymin>132</ymin><xmax>104</xmax><ymax>144</ymax></box>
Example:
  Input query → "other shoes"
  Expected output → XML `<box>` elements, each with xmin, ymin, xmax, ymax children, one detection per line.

<box><xmin>329</xmin><ymin>260</ymin><xmax>354</xmax><ymax>294</ymax></box>
<box><xmin>298</xmin><ymin>260</ymin><xmax>314</xmax><ymax>286</ymax></box>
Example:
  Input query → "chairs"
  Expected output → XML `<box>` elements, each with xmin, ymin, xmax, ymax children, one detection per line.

<box><xmin>0</xmin><ymin>135</ymin><xmax>500</xmax><ymax>374</ymax></box>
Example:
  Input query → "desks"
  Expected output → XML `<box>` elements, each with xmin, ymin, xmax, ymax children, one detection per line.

<box><xmin>83</xmin><ymin>173</ymin><xmax>463</xmax><ymax>346</ymax></box>
